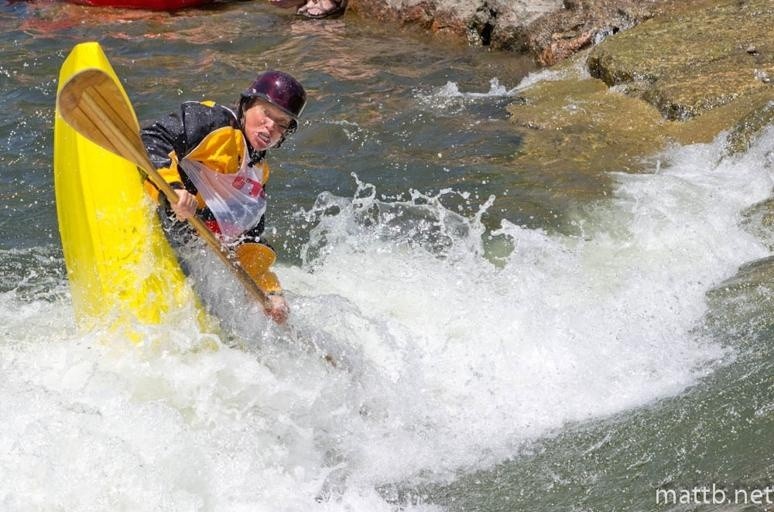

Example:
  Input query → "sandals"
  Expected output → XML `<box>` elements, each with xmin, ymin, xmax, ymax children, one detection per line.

<box><xmin>297</xmin><ymin>3</ymin><xmax>311</xmax><ymax>13</ymax></box>
<box><xmin>304</xmin><ymin>3</ymin><xmax>336</xmax><ymax>18</ymax></box>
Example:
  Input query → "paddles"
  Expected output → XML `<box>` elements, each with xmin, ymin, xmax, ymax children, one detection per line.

<box><xmin>59</xmin><ymin>68</ymin><xmax>338</xmax><ymax>367</ymax></box>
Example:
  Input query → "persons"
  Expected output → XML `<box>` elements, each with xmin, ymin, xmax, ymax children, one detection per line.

<box><xmin>138</xmin><ymin>70</ymin><xmax>309</xmax><ymax>338</ymax></box>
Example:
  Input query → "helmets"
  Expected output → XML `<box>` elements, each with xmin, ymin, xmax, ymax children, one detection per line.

<box><xmin>239</xmin><ymin>71</ymin><xmax>307</xmax><ymax>120</ymax></box>
